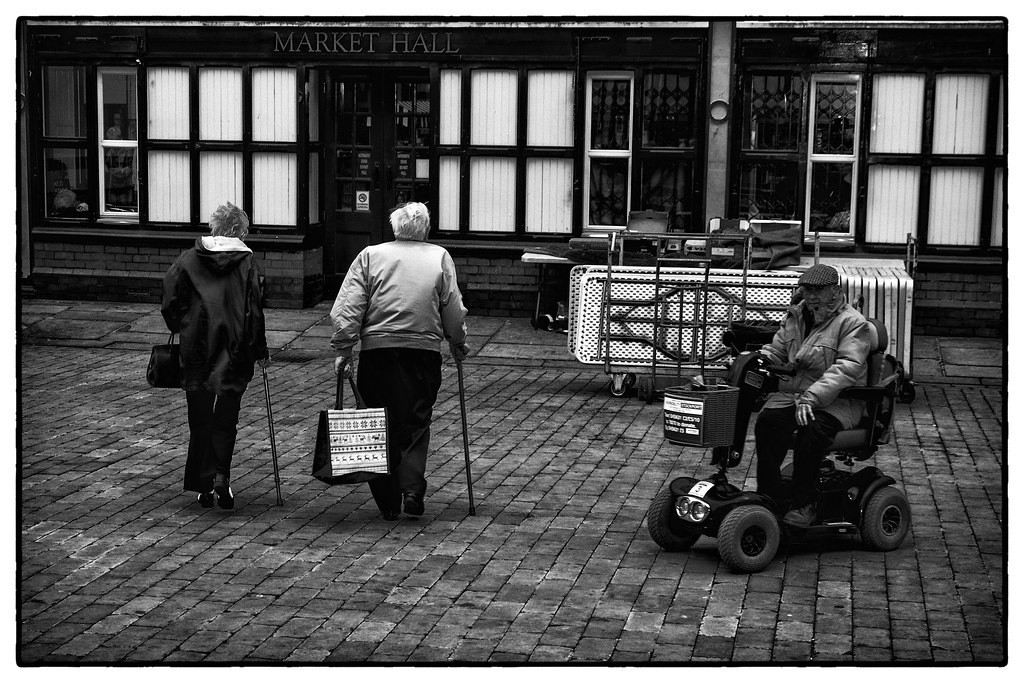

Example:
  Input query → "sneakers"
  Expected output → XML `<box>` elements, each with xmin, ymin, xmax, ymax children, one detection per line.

<box><xmin>782</xmin><ymin>501</ymin><xmax>819</xmax><ymax>526</ymax></box>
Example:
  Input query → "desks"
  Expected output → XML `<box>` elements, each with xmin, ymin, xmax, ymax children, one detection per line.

<box><xmin>520</xmin><ymin>252</ymin><xmax>599</xmax><ymax>329</ymax></box>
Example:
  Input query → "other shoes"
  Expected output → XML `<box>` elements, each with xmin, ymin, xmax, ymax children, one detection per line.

<box><xmin>382</xmin><ymin>503</ymin><xmax>399</xmax><ymax>521</ymax></box>
<box><xmin>215</xmin><ymin>485</ymin><xmax>235</xmax><ymax>510</ymax></box>
<box><xmin>403</xmin><ymin>492</ymin><xmax>426</xmax><ymax>516</ymax></box>
<box><xmin>197</xmin><ymin>489</ymin><xmax>215</xmax><ymax>508</ymax></box>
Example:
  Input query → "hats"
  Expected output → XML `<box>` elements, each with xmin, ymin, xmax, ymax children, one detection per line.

<box><xmin>798</xmin><ymin>264</ymin><xmax>838</xmax><ymax>285</ymax></box>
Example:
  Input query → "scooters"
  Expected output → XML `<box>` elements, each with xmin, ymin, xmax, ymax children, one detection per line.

<box><xmin>647</xmin><ymin>317</ymin><xmax>917</xmax><ymax>574</ymax></box>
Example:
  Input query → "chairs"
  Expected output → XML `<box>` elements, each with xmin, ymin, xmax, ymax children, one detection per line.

<box><xmin>791</xmin><ymin>318</ymin><xmax>894</xmax><ymax>450</ymax></box>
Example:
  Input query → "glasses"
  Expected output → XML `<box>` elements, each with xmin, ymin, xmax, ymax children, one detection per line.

<box><xmin>799</xmin><ymin>284</ymin><xmax>836</xmax><ymax>294</ymax></box>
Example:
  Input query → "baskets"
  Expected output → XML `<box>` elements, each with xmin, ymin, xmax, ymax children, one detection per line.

<box><xmin>664</xmin><ymin>384</ymin><xmax>739</xmax><ymax>446</ymax></box>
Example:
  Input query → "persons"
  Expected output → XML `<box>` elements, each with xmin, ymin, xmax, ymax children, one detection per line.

<box><xmin>51</xmin><ymin>126</ymin><xmax>137</xmax><ymax>213</ymax></box>
<box><xmin>161</xmin><ymin>204</ymin><xmax>272</xmax><ymax>510</ymax></box>
<box><xmin>729</xmin><ymin>264</ymin><xmax>871</xmax><ymax>528</ymax></box>
<box><xmin>329</xmin><ymin>202</ymin><xmax>471</xmax><ymax>521</ymax></box>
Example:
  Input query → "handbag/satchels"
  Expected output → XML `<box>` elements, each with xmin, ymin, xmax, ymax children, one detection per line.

<box><xmin>312</xmin><ymin>361</ymin><xmax>388</xmax><ymax>484</ymax></box>
<box><xmin>146</xmin><ymin>332</ymin><xmax>188</xmax><ymax>387</ymax></box>
